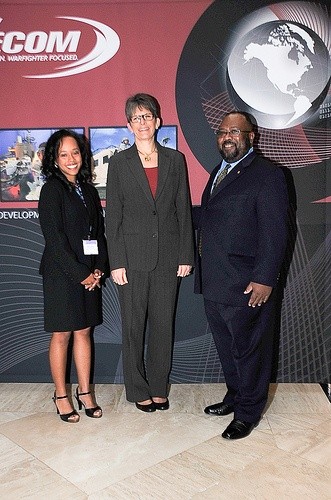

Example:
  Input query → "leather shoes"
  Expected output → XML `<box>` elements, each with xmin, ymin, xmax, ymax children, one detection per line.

<box><xmin>204</xmin><ymin>400</ymin><xmax>235</xmax><ymax>416</ymax></box>
<box><xmin>150</xmin><ymin>396</ymin><xmax>169</xmax><ymax>410</ymax></box>
<box><xmin>135</xmin><ymin>401</ymin><xmax>156</xmax><ymax>412</ymax></box>
<box><xmin>222</xmin><ymin>417</ymin><xmax>260</xmax><ymax>439</ymax></box>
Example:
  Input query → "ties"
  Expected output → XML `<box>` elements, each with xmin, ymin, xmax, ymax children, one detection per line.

<box><xmin>199</xmin><ymin>164</ymin><xmax>231</xmax><ymax>257</ymax></box>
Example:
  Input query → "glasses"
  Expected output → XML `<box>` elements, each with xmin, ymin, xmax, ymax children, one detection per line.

<box><xmin>130</xmin><ymin>114</ymin><xmax>155</xmax><ymax>123</ymax></box>
<box><xmin>215</xmin><ymin>127</ymin><xmax>251</xmax><ymax>137</ymax></box>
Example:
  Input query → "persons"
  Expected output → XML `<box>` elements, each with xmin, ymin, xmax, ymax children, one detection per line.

<box><xmin>106</xmin><ymin>93</ymin><xmax>194</xmax><ymax>412</ymax></box>
<box><xmin>196</xmin><ymin>111</ymin><xmax>298</xmax><ymax>439</ymax></box>
<box><xmin>37</xmin><ymin>130</ymin><xmax>110</xmax><ymax>423</ymax></box>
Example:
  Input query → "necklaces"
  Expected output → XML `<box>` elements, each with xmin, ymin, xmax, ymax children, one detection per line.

<box><xmin>137</xmin><ymin>149</ymin><xmax>156</xmax><ymax>161</ymax></box>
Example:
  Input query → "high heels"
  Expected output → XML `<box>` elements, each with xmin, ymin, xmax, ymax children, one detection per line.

<box><xmin>74</xmin><ymin>386</ymin><xmax>102</xmax><ymax>418</ymax></box>
<box><xmin>52</xmin><ymin>390</ymin><xmax>80</xmax><ymax>422</ymax></box>
